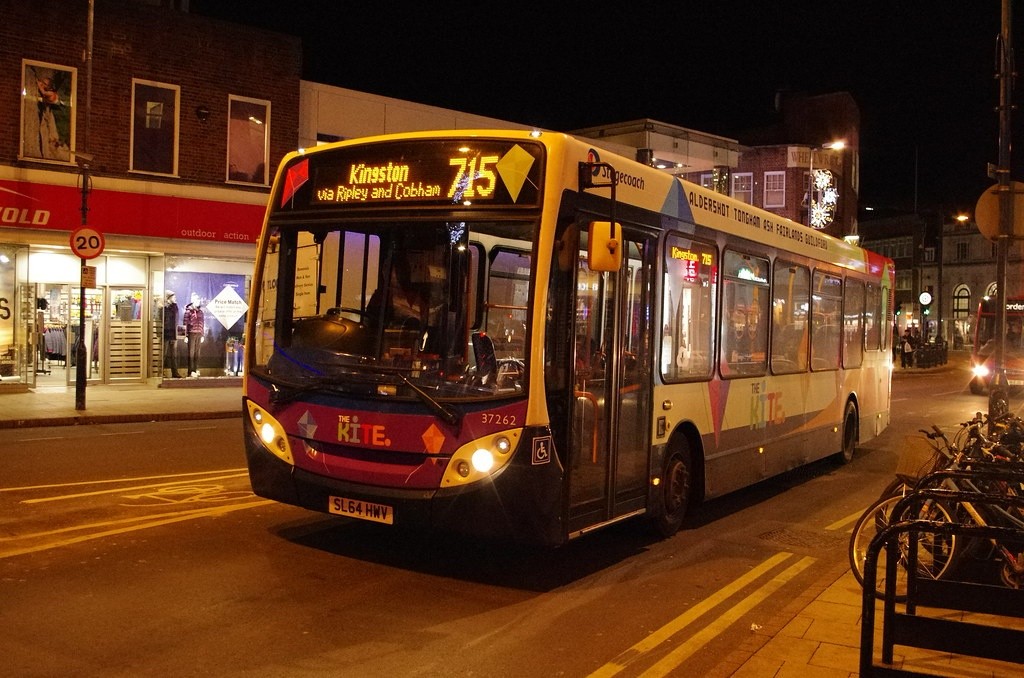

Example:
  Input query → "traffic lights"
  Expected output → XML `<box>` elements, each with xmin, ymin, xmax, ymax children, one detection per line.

<box><xmin>894</xmin><ymin>298</ymin><xmax>902</xmax><ymax>315</ymax></box>
<box><xmin>919</xmin><ymin>290</ymin><xmax>931</xmax><ymax>343</ymax></box>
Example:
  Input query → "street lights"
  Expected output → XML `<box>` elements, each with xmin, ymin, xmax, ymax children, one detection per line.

<box><xmin>807</xmin><ymin>138</ymin><xmax>845</xmax><ymax>229</ymax></box>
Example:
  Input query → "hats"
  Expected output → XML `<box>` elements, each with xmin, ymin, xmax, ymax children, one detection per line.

<box><xmin>165</xmin><ymin>290</ymin><xmax>175</xmax><ymax>299</ymax></box>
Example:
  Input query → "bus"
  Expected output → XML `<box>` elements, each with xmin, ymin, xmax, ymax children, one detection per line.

<box><xmin>241</xmin><ymin>128</ymin><xmax>896</xmax><ymax>559</ymax></box>
<box><xmin>968</xmin><ymin>294</ymin><xmax>1024</xmax><ymax>398</ymax></box>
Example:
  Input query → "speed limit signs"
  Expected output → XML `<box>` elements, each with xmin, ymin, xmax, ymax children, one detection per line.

<box><xmin>69</xmin><ymin>226</ymin><xmax>106</xmax><ymax>260</ymax></box>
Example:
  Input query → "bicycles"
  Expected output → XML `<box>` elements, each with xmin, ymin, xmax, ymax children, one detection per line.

<box><xmin>849</xmin><ymin>412</ymin><xmax>1024</xmax><ymax>617</ymax></box>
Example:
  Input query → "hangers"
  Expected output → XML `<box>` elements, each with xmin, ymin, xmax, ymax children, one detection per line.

<box><xmin>42</xmin><ymin>322</ymin><xmax>81</xmax><ymax>334</ymax></box>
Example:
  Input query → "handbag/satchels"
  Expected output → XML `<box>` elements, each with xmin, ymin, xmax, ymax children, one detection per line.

<box><xmin>905</xmin><ymin>341</ymin><xmax>912</xmax><ymax>353</ymax></box>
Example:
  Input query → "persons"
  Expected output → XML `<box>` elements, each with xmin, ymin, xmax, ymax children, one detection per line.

<box><xmin>183</xmin><ymin>294</ymin><xmax>205</xmax><ymax>378</ymax></box>
<box><xmin>35</xmin><ymin>71</ymin><xmax>70</xmax><ymax>162</ymax></box>
<box><xmin>900</xmin><ymin>330</ymin><xmax>915</xmax><ymax>369</ymax></box>
<box><xmin>155</xmin><ymin>290</ymin><xmax>183</xmax><ymax>378</ymax></box>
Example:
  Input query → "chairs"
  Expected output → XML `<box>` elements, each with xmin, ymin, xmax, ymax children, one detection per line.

<box><xmin>729</xmin><ymin>307</ymin><xmax>854</xmax><ymax>371</ymax></box>
